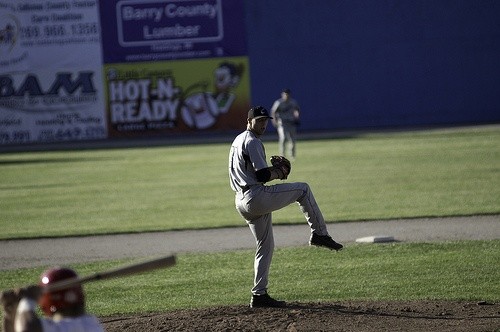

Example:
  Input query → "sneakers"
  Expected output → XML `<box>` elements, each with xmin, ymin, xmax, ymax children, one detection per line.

<box><xmin>250</xmin><ymin>294</ymin><xmax>285</xmax><ymax>308</ymax></box>
<box><xmin>310</xmin><ymin>234</ymin><xmax>343</xmax><ymax>252</ymax></box>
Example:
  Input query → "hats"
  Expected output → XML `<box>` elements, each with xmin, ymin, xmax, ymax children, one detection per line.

<box><xmin>248</xmin><ymin>106</ymin><xmax>274</xmax><ymax>120</ymax></box>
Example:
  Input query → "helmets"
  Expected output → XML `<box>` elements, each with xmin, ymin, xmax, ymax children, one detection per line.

<box><xmin>38</xmin><ymin>268</ymin><xmax>84</xmax><ymax>317</ymax></box>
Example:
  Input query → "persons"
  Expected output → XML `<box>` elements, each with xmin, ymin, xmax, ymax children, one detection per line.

<box><xmin>269</xmin><ymin>88</ymin><xmax>301</xmax><ymax>161</ymax></box>
<box><xmin>227</xmin><ymin>104</ymin><xmax>343</xmax><ymax>309</ymax></box>
<box><xmin>0</xmin><ymin>268</ymin><xmax>104</xmax><ymax>332</ymax></box>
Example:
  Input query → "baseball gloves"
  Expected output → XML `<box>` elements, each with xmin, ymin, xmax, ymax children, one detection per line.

<box><xmin>271</xmin><ymin>156</ymin><xmax>290</xmax><ymax>179</ymax></box>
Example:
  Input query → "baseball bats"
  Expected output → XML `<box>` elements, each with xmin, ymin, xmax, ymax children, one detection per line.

<box><xmin>3</xmin><ymin>255</ymin><xmax>175</xmax><ymax>305</ymax></box>
